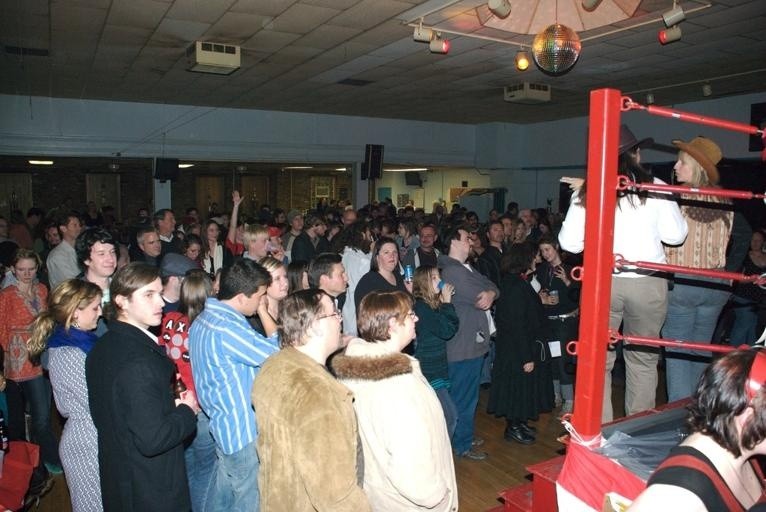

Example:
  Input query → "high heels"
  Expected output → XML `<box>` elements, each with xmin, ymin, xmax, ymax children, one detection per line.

<box><xmin>504</xmin><ymin>428</ymin><xmax>535</xmax><ymax>445</ymax></box>
<box><xmin>521</xmin><ymin>422</ymin><xmax>537</xmax><ymax>436</ymax></box>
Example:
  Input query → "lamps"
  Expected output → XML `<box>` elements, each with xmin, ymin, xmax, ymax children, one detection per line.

<box><xmin>412</xmin><ymin>17</ymin><xmax>433</xmax><ymax>43</ymax></box>
<box><xmin>515</xmin><ymin>45</ymin><xmax>531</xmax><ymax>71</ymax></box>
<box><xmin>662</xmin><ymin>2</ymin><xmax>687</xmax><ymax>27</ymax></box>
<box><xmin>533</xmin><ymin>22</ymin><xmax>581</xmax><ymax>74</ymax></box>
<box><xmin>487</xmin><ymin>0</ymin><xmax>513</xmax><ymax>20</ymax></box>
<box><xmin>660</xmin><ymin>25</ymin><xmax>683</xmax><ymax>44</ymax></box>
<box><xmin>581</xmin><ymin>0</ymin><xmax>602</xmax><ymax>12</ymax></box>
<box><xmin>702</xmin><ymin>81</ymin><xmax>715</xmax><ymax>97</ymax></box>
<box><xmin>644</xmin><ymin>91</ymin><xmax>654</xmax><ymax>104</ymax></box>
<box><xmin>430</xmin><ymin>30</ymin><xmax>450</xmax><ymax>53</ymax></box>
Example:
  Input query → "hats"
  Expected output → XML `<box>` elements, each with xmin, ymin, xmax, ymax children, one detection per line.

<box><xmin>617</xmin><ymin>124</ymin><xmax>643</xmax><ymax>157</ymax></box>
<box><xmin>158</xmin><ymin>253</ymin><xmax>203</xmax><ymax>278</ymax></box>
<box><xmin>671</xmin><ymin>135</ymin><xmax>723</xmax><ymax>187</ymax></box>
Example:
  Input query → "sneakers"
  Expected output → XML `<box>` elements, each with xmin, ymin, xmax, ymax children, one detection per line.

<box><xmin>557</xmin><ymin>400</ymin><xmax>573</xmax><ymax>421</ymax></box>
<box><xmin>553</xmin><ymin>392</ymin><xmax>563</xmax><ymax>406</ymax></box>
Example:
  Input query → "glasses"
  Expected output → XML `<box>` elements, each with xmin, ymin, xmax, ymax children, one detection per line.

<box><xmin>317</xmin><ymin>309</ymin><xmax>343</xmax><ymax>323</ymax></box>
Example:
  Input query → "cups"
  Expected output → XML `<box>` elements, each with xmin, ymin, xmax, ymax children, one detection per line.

<box><xmin>549</xmin><ymin>290</ymin><xmax>559</xmax><ymax>304</ymax></box>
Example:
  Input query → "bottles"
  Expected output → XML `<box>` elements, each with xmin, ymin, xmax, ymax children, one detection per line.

<box><xmin>405</xmin><ymin>265</ymin><xmax>413</xmax><ymax>282</ymax></box>
<box><xmin>174</xmin><ymin>374</ymin><xmax>188</xmax><ymax>400</ymax></box>
<box><xmin>0</xmin><ymin>411</ymin><xmax>8</xmax><ymax>454</ymax></box>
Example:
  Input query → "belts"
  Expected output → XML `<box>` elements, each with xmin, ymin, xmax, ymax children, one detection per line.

<box><xmin>548</xmin><ymin>309</ymin><xmax>579</xmax><ymax>320</ymax></box>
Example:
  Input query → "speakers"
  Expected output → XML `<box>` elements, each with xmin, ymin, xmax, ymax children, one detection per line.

<box><xmin>365</xmin><ymin>144</ymin><xmax>384</xmax><ymax>179</ymax></box>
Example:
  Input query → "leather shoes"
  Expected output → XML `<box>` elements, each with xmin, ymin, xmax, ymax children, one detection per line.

<box><xmin>456</xmin><ymin>449</ymin><xmax>488</xmax><ymax>460</ymax></box>
<box><xmin>472</xmin><ymin>434</ymin><xmax>485</xmax><ymax>445</ymax></box>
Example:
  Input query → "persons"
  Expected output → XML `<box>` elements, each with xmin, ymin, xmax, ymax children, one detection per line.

<box><xmin>531</xmin><ymin>234</ymin><xmax>581</xmax><ymax>420</ymax></box>
<box><xmin>189</xmin><ymin>257</ymin><xmax>280</xmax><ymax>512</ymax></box>
<box><xmin>39</xmin><ymin>223</ymin><xmax>62</xmax><ymax>270</ymax></box>
<box><xmin>76</xmin><ymin>229</ymin><xmax>120</xmax><ymax>309</ymax></box>
<box><xmin>331</xmin><ymin>290</ymin><xmax>459</xmax><ymax>512</ymax></box>
<box><xmin>355</xmin><ymin>237</ymin><xmax>415</xmax><ymax>355</ymax></box>
<box><xmin>246</xmin><ymin>204</ymin><xmax>287</xmax><ymax>225</ymax></box>
<box><xmin>404</xmin><ymin>225</ymin><xmax>440</xmax><ymax>267</ymax></box>
<box><xmin>0</xmin><ymin>240</ymin><xmax>20</xmax><ymax>291</ymax></box>
<box><xmin>558</xmin><ymin>124</ymin><xmax>688</xmax><ymax>423</ymax></box>
<box><xmin>148</xmin><ymin>253</ymin><xmax>202</xmax><ymax>336</ymax></box>
<box><xmin>487</xmin><ymin>243</ymin><xmax>556</xmax><ymax>443</ymax></box>
<box><xmin>394</xmin><ymin>219</ymin><xmax>420</xmax><ymax>261</ymax></box>
<box><xmin>159</xmin><ymin>269</ymin><xmax>212</xmax><ymax>512</ymax></box>
<box><xmin>136</xmin><ymin>208</ymin><xmax>149</xmax><ymax>225</ymax></box>
<box><xmin>0</xmin><ymin>247</ymin><xmax>65</xmax><ymax>474</ymax></box>
<box><xmin>45</xmin><ymin>200</ymin><xmax>122</xmax><ymax>227</ymax></box>
<box><xmin>285</xmin><ymin>260</ymin><xmax>310</xmax><ymax>290</ymax></box>
<box><xmin>209</xmin><ymin>203</ymin><xmax>221</xmax><ymax>218</ymax></box>
<box><xmin>181</xmin><ymin>234</ymin><xmax>203</xmax><ymax>260</ymax></box>
<box><xmin>86</xmin><ymin>261</ymin><xmax>197</xmax><ymax>512</ymax></box>
<box><xmin>135</xmin><ymin>225</ymin><xmax>161</xmax><ymax>262</ymax></box>
<box><xmin>281</xmin><ymin>209</ymin><xmax>304</xmax><ymax>264</ymax></box>
<box><xmin>728</xmin><ymin>230</ymin><xmax>766</xmax><ymax>346</ymax></box>
<box><xmin>398</xmin><ymin>199</ymin><xmax>478</xmax><ymax>226</ymax></box>
<box><xmin>319</xmin><ymin>196</ymin><xmax>398</xmax><ymax>239</ymax></box>
<box><xmin>467</xmin><ymin>232</ymin><xmax>486</xmax><ymax>266</ymax></box>
<box><xmin>342</xmin><ymin>221</ymin><xmax>376</xmax><ymax>338</ymax></box>
<box><xmin>291</xmin><ymin>214</ymin><xmax>328</xmax><ymax>263</ymax></box>
<box><xmin>46</xmin><ymin>212</ymin><xmax>81</xmax><ymax>290</ymax></box>
<box><xmin>627</xmin><ymin>350</ymin><xmax>766</xmax><ymax>512</ymax></box>
<box><xmin>489</xmin><ymin>201</ymin><xmax>565</xmax><ymax>239</ymax></box>
<box><xmin>154</xmin><ymin>209</ymin><xmax>184</xmax><ymax>257</ymax></box>
<box><xmin>178</xmin><ymin>206</ymin><xmax>202</xmax><ymax>233</ymax></box>
<box><xmin>224</xmin><ymin>190</ymin><xmax>245</xmax><ymax>257</ymax></box>
<box><xmin>245</xmin><ymin>256</ymin><xmax>288</xmax><ymax>338</ymax></box>
<box><xmin>251</xmin><ymin>289</ymin><xmax>373</xmax><ymax>512</ymax></box>
<box><xmin>414</xmin><ymin>265</ymin><xmax>459</xmax><ymax>444</ymax></box>
<box><xmin>27</xmin><ymin>279</ymin><xmax>102</xmax><ymax>512</ymax></box>
<box><xmin>243</xmin><ymin>224</ymin><xmax>269</xmax><ymax>262</ymax></box>
<box><xmin>0</xmin><ymin>207</ymin><xmax>44</xmax><ymax>247</ymax></box>
<box><xmin>308</xmin><ymin>251</ymin><xmax>355</xmax><ymax>376</ymax></box>
<box><xmin>441</xmin><ymin>227</ymin><xmax>500</xmax><ymax>459</ymax></box>
<box><xmin>661</xmin><ymin>135</ymin><xmax>752</xmax><ymax>401</ymax></box>
<box><xmin>201</xmin><ymin>221</ymin><xmax>223</xmax><ymax>296</ymax></box>
<box><xmin>474</xmin><ymin>222</ymin><xmax>505</xmax><ymax>278</ymax></box>
